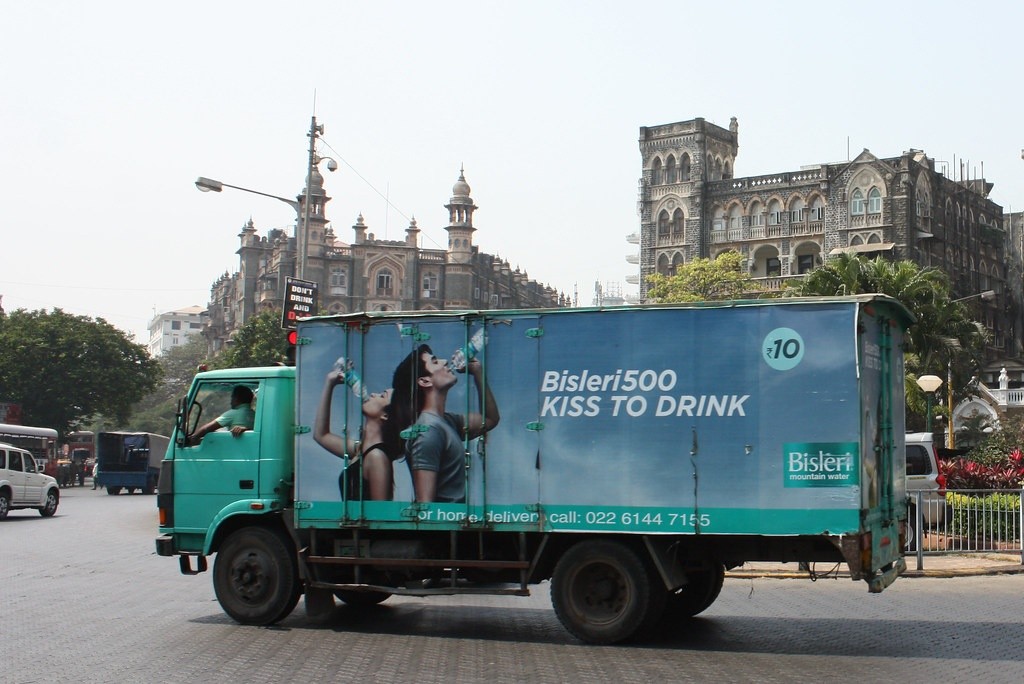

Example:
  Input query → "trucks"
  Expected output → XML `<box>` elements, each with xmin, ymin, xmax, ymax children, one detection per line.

<box><xmin>152</xmin><ymin>290</ymin><xmax>917</xmax><ymax>646</ymax></box>
<box><xmin>95</xmin><ymin>428</ymin><xmax>171</xmax><ymax>495</ymax></box>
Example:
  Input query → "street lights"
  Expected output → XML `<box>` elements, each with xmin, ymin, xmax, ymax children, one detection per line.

<box><xmin>915</xmin><ymin>373</ymin><xmax>944</xmax><ymax>432</ymax></box>
<box><xmin>192</xmin><ymin>116</ymin><xmax>337</xmax><ymax>278</ymax></box>
<box><xmin>948</xmin><ymin>289</ymin><xmax>997</xmax><ymax>304</ymax></box>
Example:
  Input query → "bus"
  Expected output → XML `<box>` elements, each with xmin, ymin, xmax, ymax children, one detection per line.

<box><xmin>0</xmin><ymin>424</ymin><xmax>59</xmax><ymax>479</ymax></box>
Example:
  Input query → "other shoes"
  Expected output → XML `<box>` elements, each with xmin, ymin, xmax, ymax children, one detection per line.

<box><xmin>79</xmin><ymin>484</ymin><xmax>84</xmax><ymax>486</ymax></box>
<box><xmin>91</xmin><ymin>488</ymin><xmax>95</xmax><ymax>490</ymax></box>
<box><xmin>101</xmin><ymin>486</ymin><xmax>103</xmax><ymax>489</ymax></box>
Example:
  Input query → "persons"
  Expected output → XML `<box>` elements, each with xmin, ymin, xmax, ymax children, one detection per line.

<box><xmin>57</xmin><ymin>463</ymin><xmax>68</xmax><ymax>488</ymax></box>
<box><xmin>313</xmin><ymin>344</ymin><xmax>500</xmax><ymax>503</ymax></box>
<box><xmin>91</xmin><ymin>459</ymin><xmax>103</xmax><ymax>489</ymax></box>
<box><xmin>70</xmin><ymin>458</ymin><xmax>86</xmax><ymax>487</ymax></box>
<box><xmin>187</xmin><ymin>385</ymin><xmax>255</xmax><ymax>445</ymax></box>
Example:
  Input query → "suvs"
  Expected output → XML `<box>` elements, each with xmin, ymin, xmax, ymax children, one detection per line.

<box><xmin>903</xmin><ymin>430</ymin><xmax>948</xmax><ymax>552</ymax></box>
<box><xmin>0</xmin><ymin>442</ymin><xmax>62</xmax><ymax>520</ymax></box>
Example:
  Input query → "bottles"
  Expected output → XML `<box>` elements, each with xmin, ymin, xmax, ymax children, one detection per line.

<box><xmin>449</xmin><ymin>328</ymin><xmax>490</xmax><ymax>371</ymax></box>
<box><xmin>332</xmin><ymin>357</ymin><xmax>370</xmax><ymax>402</ymax></box>
